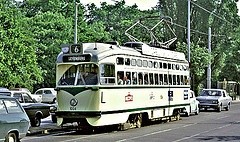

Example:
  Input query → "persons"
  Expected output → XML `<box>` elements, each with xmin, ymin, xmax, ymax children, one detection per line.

<box><xmin>84</xmin><ymin>64</ymin><xmax>130</xmax><ymax>85</ymax></box>
<box><xmin>232</xmin><ymin>81</ymin><xmax>240</xmax><ymax>100</ymax></box>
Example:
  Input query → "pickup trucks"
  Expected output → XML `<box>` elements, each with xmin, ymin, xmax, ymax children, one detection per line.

<box><xmin>10</xmin><ymin>88</ymin><xmax>42</xmax><ymax>103</ymax></box>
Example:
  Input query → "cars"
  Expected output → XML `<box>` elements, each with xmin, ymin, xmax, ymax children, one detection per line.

<box><xmin>0</xmin><ymin>96</ymin><xmax>30</xmax><ymax>142</ymax></box>
<box><xmin>180</xmin><ymin>90</ymin><xmax>200</xmax><ymax>117</ymax></box>
<box><xmin>0</xmin><ymin>90</ymin><xmax>57</xmax><ymax>127</ymax></box>
<box><xmin>35</xmin><ymin>88</ymin><xmax>57</xmax><ymax>104</ymax></box>
<box><xmin>196</xmin><ymin>89</ymin><xmax>232</xmax><ymax>113</ymax></box>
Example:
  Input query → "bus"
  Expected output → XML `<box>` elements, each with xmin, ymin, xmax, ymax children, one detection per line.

<box><xmin>55</xmin><ymin>40</ymin><xmax>191</xmax><ymax>131</ymax></box>
